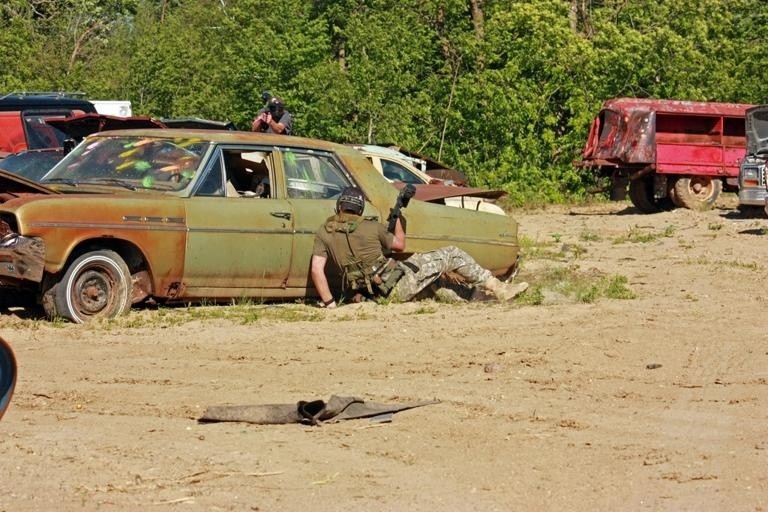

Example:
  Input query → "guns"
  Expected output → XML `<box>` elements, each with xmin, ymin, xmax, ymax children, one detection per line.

<box><xmin>260</xmin><ymin>92</ymin><xmax>270</xmax><ymax>130</ymax></box>
<box><xmin>387</xmin><ymin>185</ymin><xmax>416</xmax><ymax>235</ymax></box>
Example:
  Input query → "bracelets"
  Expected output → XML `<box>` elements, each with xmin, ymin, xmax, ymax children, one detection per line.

<box><xmin>324</xmin><ymin>296</ymin><xmax>336</xmax><ymax>307</ymax></box>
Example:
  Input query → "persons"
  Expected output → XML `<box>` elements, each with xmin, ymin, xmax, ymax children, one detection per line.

<box><xmin>309</xmin><ymin>185</ymin><xmax>530</xmax><ymax>311</ymax></box>
<box><xmin>251</xmin><ymin>97</ymin><xmax>293</xmax><ymax>136</ymax></box>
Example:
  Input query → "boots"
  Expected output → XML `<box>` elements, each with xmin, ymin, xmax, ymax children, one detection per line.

<box><xmin>488</xmin><ymin>278</ymin><xmax>529</xmax><ymax>302</ymax></box>
<box><xmin>470</xmin><ymin>288</ymin><xmax>495</xmax><ymax>302</ymax></box>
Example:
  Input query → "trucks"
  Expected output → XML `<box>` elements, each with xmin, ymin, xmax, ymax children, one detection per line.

<box><xmin>572</xmin><ymin>96</ymin><xmax>761</xmax><ymax>212</ymax></box>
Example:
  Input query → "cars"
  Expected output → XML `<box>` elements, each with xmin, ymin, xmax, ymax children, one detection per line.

<box><xmin>0</xmin><ymin>88</ymin><xmax>521</xmax><ymax>326</ymax></box>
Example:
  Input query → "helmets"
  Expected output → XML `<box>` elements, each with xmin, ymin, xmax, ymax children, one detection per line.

<box><xmin>270</xmin><ymin>97</ymin><xmax>284</xmax><ymax>117</ymax></box>
<box><xmin>337</xmin><ymin>187</ymin><xmax>364</xmax><ymax>213</ymax></box>
